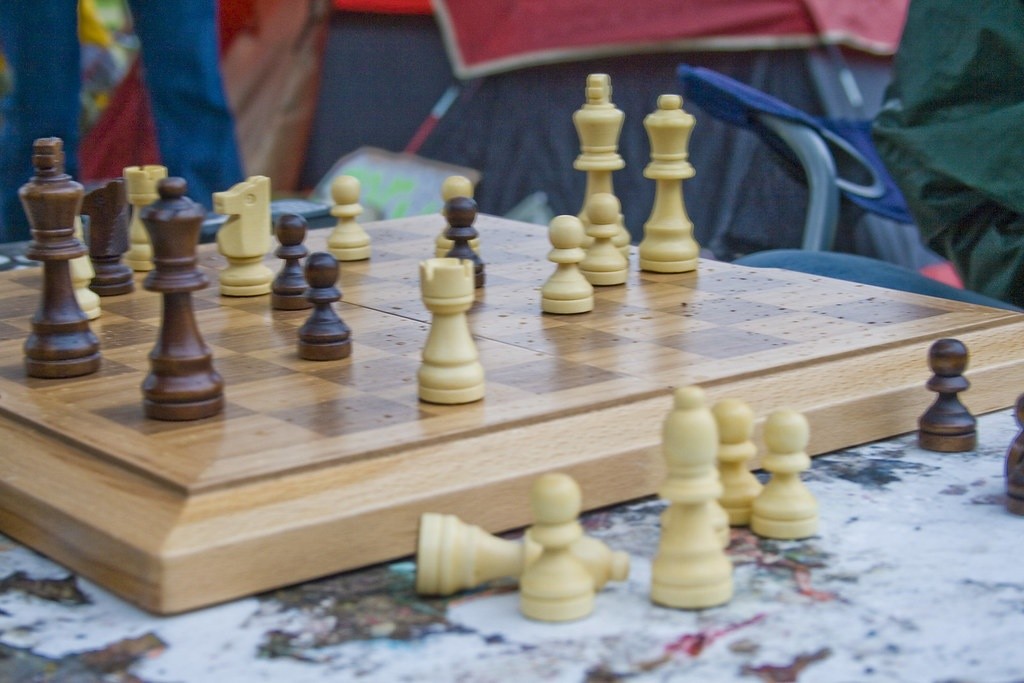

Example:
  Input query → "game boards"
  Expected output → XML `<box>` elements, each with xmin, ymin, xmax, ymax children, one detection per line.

<box><xmin>0</xmin><ymin>207</ymin><xmax>1023</xmax><ymax>618</ymax></box>
